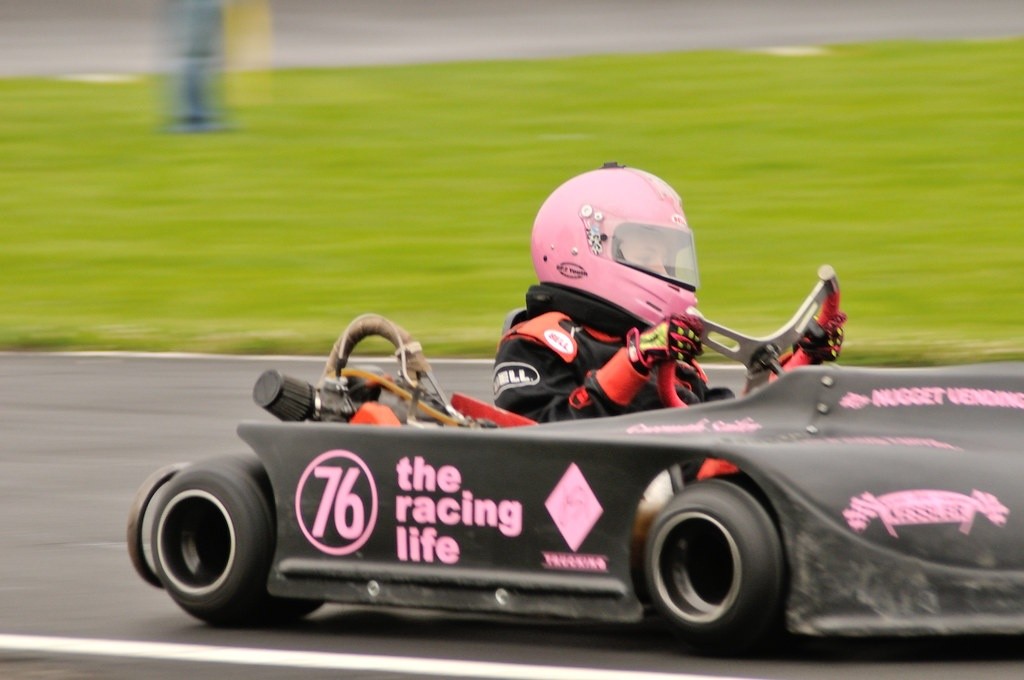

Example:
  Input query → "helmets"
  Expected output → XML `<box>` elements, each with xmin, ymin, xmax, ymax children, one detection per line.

<box><xmin>530</xmin><ymin>161</ymin><xmax>701</xmax><ymax>326</ymax></box>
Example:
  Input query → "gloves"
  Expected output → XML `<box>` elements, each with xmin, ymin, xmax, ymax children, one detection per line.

<box><xmin>794</xmin><ymin>317</ymin><xmax>843</xmax><ymax>361</ymax></box>
<box><xmin>627</xmin><ymin>317</ymin><xmax>705</xmax><ymax>375</ymax></box>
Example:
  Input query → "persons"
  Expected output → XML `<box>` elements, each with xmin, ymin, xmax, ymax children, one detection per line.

<box><xmin>493</xmin><ymin>165</ymin><xmax>846</xmax><ymax>424</ymax></box>
